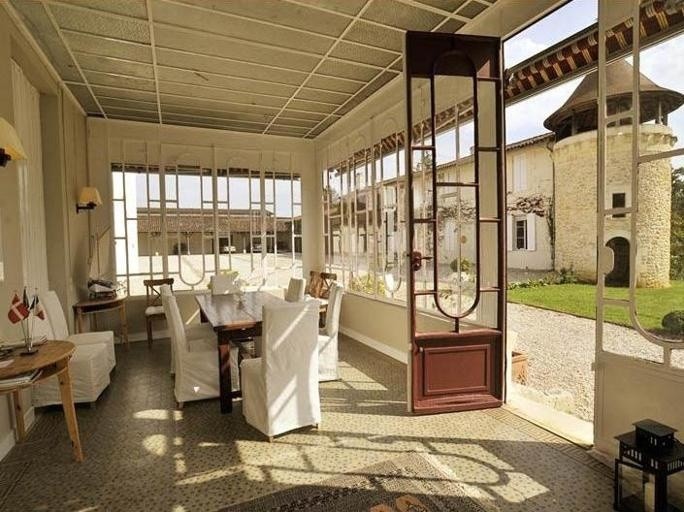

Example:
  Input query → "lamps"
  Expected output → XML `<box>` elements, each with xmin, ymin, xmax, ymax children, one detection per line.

<box><xmin>0</xmin><ymin>116</ymin><xmax>30</xmax><ymax>167</ymax></box>
<box><xmin>75</xmin><ymin>186</ymin><xmax>102</xmax><ymax>214</ymax></box>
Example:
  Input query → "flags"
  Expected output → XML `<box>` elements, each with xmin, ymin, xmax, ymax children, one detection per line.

<box><xmin>7</xmin><ymin>293</ymin><xmax>31</xmax><ymax>325</ymax></box>
<box><xmin>23</xmin><ymin>288</ymin><xmax>31</xmax><ymax>321</ymax></box>
<box><xmin>30</xmin><ymin>295</ymin><xmax>46</xmax><ymax>320</ymax></box>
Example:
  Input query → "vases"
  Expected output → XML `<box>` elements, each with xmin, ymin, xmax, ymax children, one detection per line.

<box><xmin>512</xmin><ymin>349</ymin><xmax>529</xmax><ymax>385</ymax></box>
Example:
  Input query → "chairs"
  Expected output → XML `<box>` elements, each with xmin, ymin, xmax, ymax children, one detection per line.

<box><xmin>21</xmin><ymin>296</ymin><xmax>111</xmax><ymax>410</ymax></box>
<box><xmin>210</xmin><ymin>274</ymin><xmax>235</xmax><ymax>294</ymax></box>
<box><xmin>319</xmin><ymin>272</ymin><xmax>337</xmax><ymax>327</ymax></box>
<box><xmin>163</xmin><ymin>291</ymin><xmax>239</xmax><ymax>410</ymax></box>
<box><xmin>307</xmin><ymin>270</ymin><xmax>321</xmax><ymax>299</ymax></box>
<box><xmin>160</xmin><ymin>284</ymin><xmax>217</xmax><ymax>378</ymax></box>
<box><xmin>318</xmin><ymin>280</ymin><xmax>345</xmax><ymax>383</ymax></box>
<box><xmin>143</xmin><ymin>278</ymin><xmax>174</xmax><ymax>347</ymax></box>
<box><xmin>39</xmin><ymin>290</ymin><xmax>117</xmax><ymax>374</ymax></box>
<box><xmin>253</xmin><ymin>277</ymin><xmax>306</xmax><ymax>357</ymax></box>
<box><xmin>240</xmin><ymin>299</ymin><xmax>320</xmax><ymax>443</ymax></box>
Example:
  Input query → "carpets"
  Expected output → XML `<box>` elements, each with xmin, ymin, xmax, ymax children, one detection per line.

<box><xmin>211</xmin><ymin>448</ymin><xmax>501</xmax><ymax>512</ymax></box>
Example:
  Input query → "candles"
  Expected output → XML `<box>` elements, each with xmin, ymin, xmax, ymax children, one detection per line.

<box><xmin>644</xmin><ymin>483</ymin><xmax>655</xmax><ymax>511</ymax></box>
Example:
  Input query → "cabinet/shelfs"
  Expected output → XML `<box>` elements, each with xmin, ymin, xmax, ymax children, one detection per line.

<box><xmin>613</xmin><ymin>417</ymin><xmax>683</xmax><ymax>512</ymax></box>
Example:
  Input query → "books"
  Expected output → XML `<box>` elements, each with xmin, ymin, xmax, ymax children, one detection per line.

<box><xmin>0</xmin><ymin>368</ymin><xmax>44</xmax><ymax>390</ymax></box>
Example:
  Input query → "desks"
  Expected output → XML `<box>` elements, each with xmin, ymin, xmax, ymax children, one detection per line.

<box><xmin>193</xmin><ymin>292</ymin><xmax>288</xmax><ymax>414</ymax></box>
<box><xmin>0</xmin><ymin>339</ymin><xmax>85</xmax><ymax>464</ymax></box>
<box><xmin>72</xmin><ymin>292</ymin><xmax>129</xmax><ymax>352</ymax></box>
